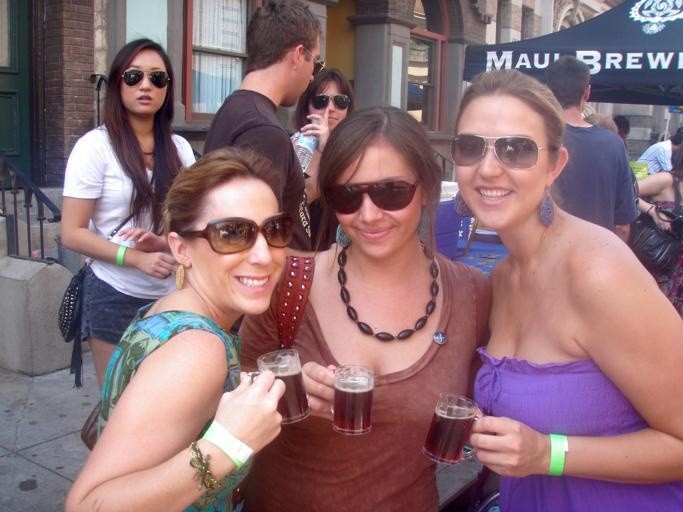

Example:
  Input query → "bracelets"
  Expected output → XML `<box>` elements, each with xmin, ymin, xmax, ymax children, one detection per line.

<box><xmin>204</xmin><ymin>422</ymin><xmax>252</xmax><ymax>468</ymax></box>
<box><xmin>547</xmin><ymin>433</ymin><xmax>570</xmax><ymax>478</ymax></box>
<box><xmin>187</xmin><ymin>442</ymin><xmax>223</xmax><ymax>499</ymax></box>
<box><xmin>114</xmin><ymin>244</ymin><xmax>126</xmax><ymax>267</ymax></box>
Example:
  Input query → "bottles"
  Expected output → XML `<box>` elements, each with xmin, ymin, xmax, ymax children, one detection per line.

<box><xmin>292</xmin><ymin>117</ymin><xmax>320</xmax><ymax>172</ymax></box>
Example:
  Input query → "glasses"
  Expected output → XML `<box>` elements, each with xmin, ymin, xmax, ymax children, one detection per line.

<box><xmin>179</xmin><ymin>212</ymin><xmax>295</xmax><ymax>254</ymax></box>
<box><xmin>304</xmin><ymin>46</ymin><xmax>326</xmax><ymax>74</ymax></box>
<box><xmin>451</xmin><ymin>133</ymin><xmax>559</xmax><ymax>170</ymax></box>
<box><xmin>312</xmin><ymin>95</ymin><xmax>351</xmax><ymax>110</ymax></box>
<box><xmin>322</xmin><ymin>180</ymin><xmax>423</xmax><ymax>214</ymax></box>
<box><xmin>121</xmin><ymin>69</ymin><xmax>172</xmax><ymax>88</ymax></box>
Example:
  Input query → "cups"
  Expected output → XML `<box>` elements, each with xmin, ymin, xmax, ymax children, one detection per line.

<box><xmin>421</xmin><ymin>392</ymin><xmax>484</xmax><ymax>466</ymax></box>
<box><xmin>327</xmin><ymin>365</ymin><xmax>373</xmax><ymax>436</ymax></box>
<box><xmin>244</xmin><ymin>347</ymin><xmax>312</xmax><ymax>426</ymax></box>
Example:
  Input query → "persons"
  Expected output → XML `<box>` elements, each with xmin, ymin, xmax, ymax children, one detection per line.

<box><xmin>454</xmin><ymin>55</ymin><xmax>638</xmax><ymax>243</ymax></box>
<box><xmin>201</xmin><ymin>0</ymin><xmax>327</xmax><ymax>249</ymax></box>
<box><xmin>60</xmin><ymin>148</ymin><xmax>287</xmax><ymax>512</ymax></box>
<box><xmin>449</xmin><ymin>72</ymin><xmax>681</xmax><ymax>511</ymax></box>
<box><xmin>234</xmin><ymin>103</ymin><xmax>494</xmax><ymax>512</ymax></box>
<box><xmin>55</xmin><ymin>39</ymin><xmax>197</xmax><ymax>396</ymax></box>
<box><xmin>290</xmin><ymin>67</ymin><xmax>355</xmax><ymax>249</ymax></box>
<box><xmin>580</xmin><ymin>104</ymin><xmax>681</xmax><ymax>327</ymax></box>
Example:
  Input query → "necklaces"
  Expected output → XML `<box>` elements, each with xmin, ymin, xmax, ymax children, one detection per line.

<box><xmin>335</xmin><ymin>239</ymin><xmax>441</xmax><ymax>340</ymax></box>
<box><xmin>143</xmin><ymin>149</ymin><xmax>154</xmax><ymax>159</ymax></box>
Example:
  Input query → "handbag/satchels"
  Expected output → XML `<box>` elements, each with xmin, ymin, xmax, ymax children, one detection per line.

<box><xmin>629</xmin><ymin>205</ymin><xmax>683</xmax><ymax>273</ymax></box>
<box><xmin>58</xmin><ymin>268</ymin><xmax>87</xmax><ymax>341</ymax></box>
